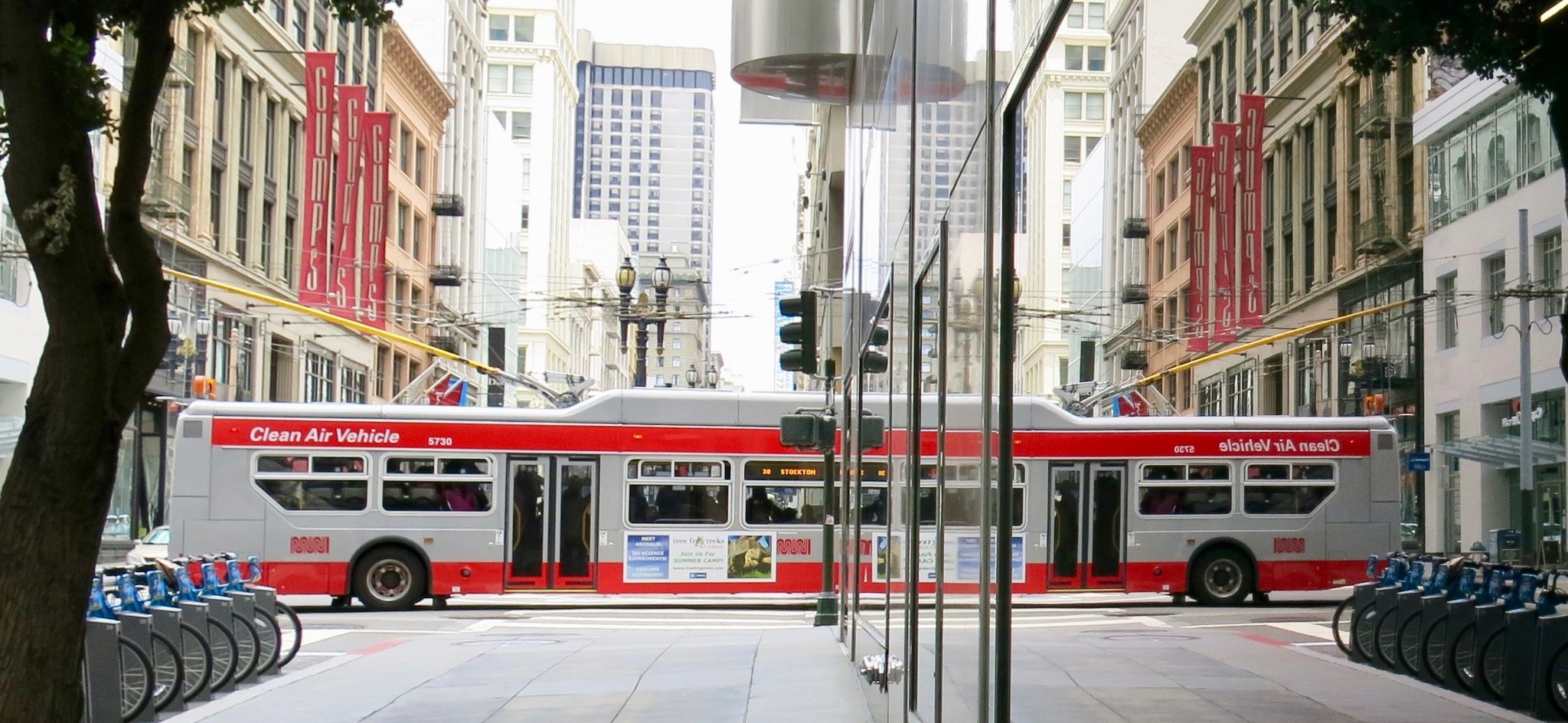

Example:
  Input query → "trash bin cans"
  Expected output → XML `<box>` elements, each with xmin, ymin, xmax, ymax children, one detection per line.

<box><xmin>1490</xmin><ymin>528</ymin><xmax>1521</xmax><ymax>565</ymax></box>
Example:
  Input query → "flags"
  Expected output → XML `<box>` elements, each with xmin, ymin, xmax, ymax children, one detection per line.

<box><xmin>1112</xmin><ymin>370</ymin><xmax>1171</xmax><ymax>418</ymax></box>
<box><xmin>405</xmin><ymin>360</ymin><xmax>470</xmax><ymax>407</ymax></box>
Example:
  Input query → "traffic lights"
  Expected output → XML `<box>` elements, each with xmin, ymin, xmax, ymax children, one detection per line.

<box><xmin>779</xmin><ymin>290</ymin><xmax>817</xmax><ymax>374</ymax></box>
<box><xmin>860</xmin><ymin>294</ymin><xmax>890</xmax><ymax>377</ymax></box>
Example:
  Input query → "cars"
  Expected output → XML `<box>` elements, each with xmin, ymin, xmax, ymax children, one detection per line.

<box><xmin>126</xmin><ymin>525</ymin><xmax>171</xmax><ymax>566</ymax></box>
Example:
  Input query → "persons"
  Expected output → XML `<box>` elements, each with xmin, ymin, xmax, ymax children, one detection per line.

<box><xmin>1140</xmin><ymin>466</ymin><xmax>1324</xmax><ymax>513</ymax></box>
<box><xmin>630</xmin><ymin>486</ymin><xmax>728</xmax><ymax>524</ymax></box>
<box><xmin>1054</xmin><ymin>476</ymin><xmax>1120</xmax><ymax>577</ymax></box>
<box><xmin>262</xmin><ymin>458</ymin><xmax>491</xmax><ymax>512</ymax></box>
<box><xmin>514</xmin><ymin>465</ymin><xmax>591</xmax><ymax>548</ymax></box>
<box><xmin>747</xmin><ymin>483</ymin><xmax>1024</xmax><ymax>524</ymax></box>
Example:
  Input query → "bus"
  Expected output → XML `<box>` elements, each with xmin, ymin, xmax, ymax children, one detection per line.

<box><xmin>866</xmin><ymin>287</ymin><xmax>1441</xmax><ymax>610</ymax></box>
<box><xmin>162</xmin><ymin>264</ymin><xmax>828</xmax><ymax>607</ymax></box>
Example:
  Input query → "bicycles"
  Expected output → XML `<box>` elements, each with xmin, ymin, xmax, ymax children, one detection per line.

<box><xmin>80</xmin><ymin>552</ymin><xmax>302</xmax><ymax>722</ymax></box>
<box><xmin>1330</xmin><ymin>550</ymin><xmax>1568</xmax><ymax>723</ymax></box>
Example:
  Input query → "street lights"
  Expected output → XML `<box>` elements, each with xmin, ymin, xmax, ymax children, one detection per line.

<box><xmin>617</xmin><ymin>255</ymin><xmax>672</xmax><ymax>387</ymax></box>
<box><xmin>947</xmin><ymin>265</ymin><xmax>1022</xmax><ymax>393</ymax></box>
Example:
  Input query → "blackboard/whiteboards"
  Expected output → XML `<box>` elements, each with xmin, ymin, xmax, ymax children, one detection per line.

<box><xmin>1538</xmin><ymin>541</ymin><xmax>1561</xmax><ymax>565</ymax></box>
